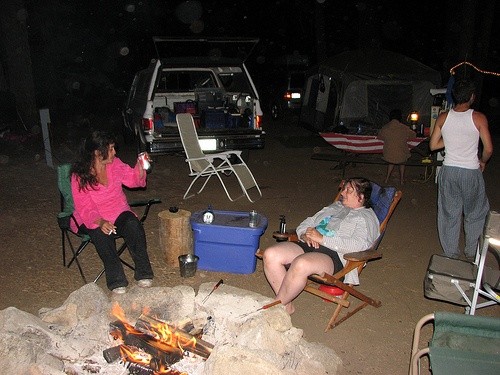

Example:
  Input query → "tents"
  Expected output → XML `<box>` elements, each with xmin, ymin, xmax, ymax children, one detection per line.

<box><xmin>297</xmin><ymin>47</ymin><xmax>442</xmax><ymax>136</ymax></box>
<box><xmin>439</xmin><ymin>56</ymin><xmax>487</xmax><ymax>112</ymax></box>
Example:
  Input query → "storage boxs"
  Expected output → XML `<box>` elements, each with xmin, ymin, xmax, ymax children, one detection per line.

<box><xmin>202</xmin><ymin>109</ymin><xmax>225</xmax><ymax>128</ymax></box>
<box><xmin>190</xmin><ymin>209</ymin><xmax>269</xmax><ymax>282</ymax></box>
<box><xmin>173</xmin><ymin>102</ymin><xmax>198</xmax><ymax>114</ymax></box>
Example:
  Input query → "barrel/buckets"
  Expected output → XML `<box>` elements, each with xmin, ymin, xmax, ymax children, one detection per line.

<box><xmin>178</xmin><ymin>254</ymin><xmax>199</xmax><ymax>277</ymax></box>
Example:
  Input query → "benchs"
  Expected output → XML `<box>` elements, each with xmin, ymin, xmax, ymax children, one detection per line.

<box><xmin>310</xmin><ymin>152</ymin><xmax>442</xmax><ymax>183</ymax></box>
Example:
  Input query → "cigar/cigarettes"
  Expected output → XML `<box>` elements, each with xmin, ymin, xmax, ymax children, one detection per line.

<box><xmin>108</xmin><ymin>230</ymin><xmax>112</xmax><ymax>236</ymax></box>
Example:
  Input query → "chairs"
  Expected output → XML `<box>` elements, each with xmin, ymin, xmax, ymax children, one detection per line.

<box><xmin>53</xmin><ymin>161</ymin><xmax>163</xmax><ymax>285</ymax></box>
<box><xmin>408</xmin><ymin>311</ymin><xmax>500</xmax><ymax>375</ymax></box>
<box><xmin>174</xmin><ymin>112</ymin><xmax>264</xmax><ymax>204</ymax></box>
<box><xmin>254</xmin><ymin>182</ymin><xmax>404</xmax><ymax>335</ymax></box>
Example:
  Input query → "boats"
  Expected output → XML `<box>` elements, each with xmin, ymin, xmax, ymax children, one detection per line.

<box><xmin>319</xmin><ymin>130</ymin><xmax>427</xmax><ymax>153</ymax></box>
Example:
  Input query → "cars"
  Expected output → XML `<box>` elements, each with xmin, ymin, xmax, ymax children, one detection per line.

<box><xmin>259</xmin><ymin>70</ymin><xmax>311</xmax><ymax>121</ymax></box>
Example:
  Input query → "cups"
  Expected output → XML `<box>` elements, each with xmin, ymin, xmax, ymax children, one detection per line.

<box><xmin>249</xmin><ymin>209</ymin><xmax>258</xmax><ymax>228</ymax></box>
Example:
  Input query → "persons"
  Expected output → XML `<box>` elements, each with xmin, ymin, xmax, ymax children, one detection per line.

<box><xmin>377</xmin><ymin>109</ymin><xmax>416</xmax><ymax>187</ymax></box>
<box><xmin>429</xmin><ymin>78</ymin><xmax>493</xmax><ymax>264</ymax></box>
<box><xmin>263</xmin><ymin>177</ymin><xmax>381</xmax><ymax>315</ymax></box>
<box><xmin>69</xmin><ymin>130</ymin><xmax>154</xmax><ymax>294</ymax></box>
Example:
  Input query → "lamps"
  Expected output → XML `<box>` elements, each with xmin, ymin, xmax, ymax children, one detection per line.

<box><xmin>405</xmin><ymin>106</ymin><xmax>420</xmax><ymax>126</ymax></box>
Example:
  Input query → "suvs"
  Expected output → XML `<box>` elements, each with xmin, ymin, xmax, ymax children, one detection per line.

<box><xmin>121</xmin><ymin>34</ymin><xmax>267</xmax><ymax>159</ymax></box>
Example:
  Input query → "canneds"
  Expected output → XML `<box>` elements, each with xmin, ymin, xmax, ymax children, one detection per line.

<box><xmin>138</xmin><ymin>152</ymin><xmax>151</xmax><ymax>170</ymax></box>
<box><xmin>248</xmin><ymin>209</ymin><xmax>257</xmax><ymax>228</ymax></box>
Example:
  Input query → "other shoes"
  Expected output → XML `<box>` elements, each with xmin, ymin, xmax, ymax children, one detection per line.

<box><xmin>137</xmin><ymin>279</ymin><xmax>153</xmax><ymax>289</ymax></box>
<box><xmin>112</xmin><ymin>286</ymin><xmax>128</xmax><ymax>294</ymax></box>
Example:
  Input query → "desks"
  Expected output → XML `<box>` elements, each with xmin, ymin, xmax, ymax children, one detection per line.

<box><xmin>317</xmin><ymin>126</ymin><xmax>433</xmax><ymax>180</ymax></box>
